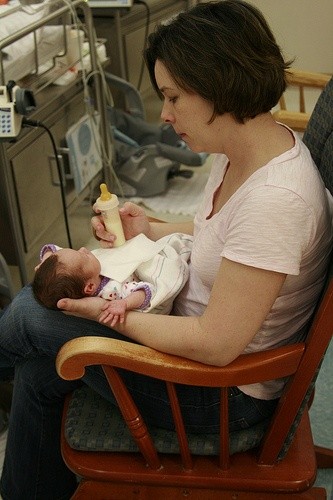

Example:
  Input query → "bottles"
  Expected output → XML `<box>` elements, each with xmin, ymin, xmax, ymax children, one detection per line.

<box><xmin>95</xmin><ymin>184</ymin><xmax>126</xmax><ymax>248</ymax></box>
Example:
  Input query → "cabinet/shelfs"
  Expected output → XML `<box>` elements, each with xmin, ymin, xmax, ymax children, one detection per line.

<box><xmin>0</xmin><ymin>57</ymin><xmax>111</xmax><ymax>288</ymax></box>
<box><xmin>74</xmin><ymin>0</ymin><xmax>194</xmax><ymax>124</ymax></box>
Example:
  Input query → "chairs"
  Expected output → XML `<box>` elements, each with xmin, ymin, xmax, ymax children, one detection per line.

<box><xmin>56</xmin><ymin>272</ymin><xmax>332</xmax><ymax>500</ymax></box>
<box><xmin>272</xmin><ymin>69</ymin><xmax>332</xmax><ymax>131</ymax></box>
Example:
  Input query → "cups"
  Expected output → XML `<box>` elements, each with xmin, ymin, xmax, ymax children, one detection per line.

<box><xmin>65</xmin><ymin>30</ymin><xmax>84</xmax><ymax>65</ymax></box>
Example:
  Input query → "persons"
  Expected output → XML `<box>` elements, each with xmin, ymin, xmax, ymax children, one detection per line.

<box><xmin>33</xmin><ymin>232</ymin><xmax>194</xmax><ymax>327</ymax></box>
<box><xmin>0</xmin><ymin>0</ymin><xmax>332</xmax><ymax>500</ymax></box>
